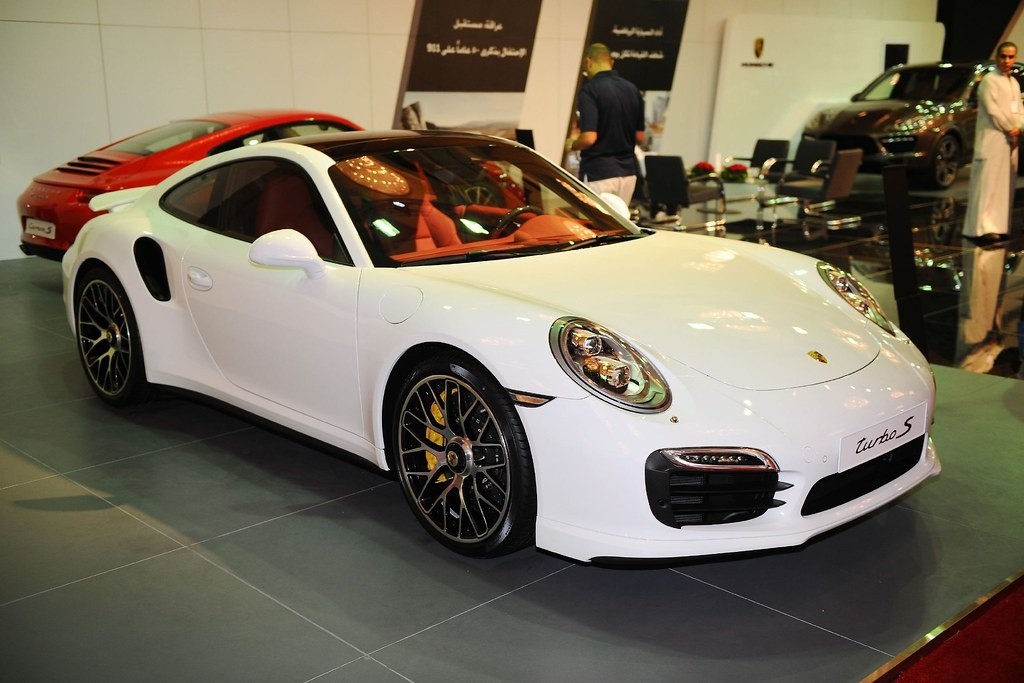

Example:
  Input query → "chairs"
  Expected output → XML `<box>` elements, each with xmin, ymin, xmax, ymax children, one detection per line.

<box><xmin>255</xmin><ymin>175</ymin><xmax>334</xmax><ymax>258</ymax></box>
<box><xmin>370</xmin><ymin>160</ymin><xmax>462</xmax><ymax>256</ymax></box>
<box><xmin>725</xmin><ymin>139</ymin><xmax>863</xmax><ymax>226</ymax></box>
<box><xmin>633</xmin><ymin>154</ymin><xmax>649</xmax><ymax>208</ymax></box>
<box><xmin>645</xmin><ymin>155</ymin><xmax>726</xmax><ymax>232</ymax></box>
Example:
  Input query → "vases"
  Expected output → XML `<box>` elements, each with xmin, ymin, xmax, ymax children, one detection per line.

<box><xmin>724</xmin><ymin>174</ymin><xmax>745</xmax><ymax>183</ymax></box>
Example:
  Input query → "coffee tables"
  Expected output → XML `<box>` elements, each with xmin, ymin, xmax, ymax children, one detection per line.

<box><xmin>689</xmin><ymin>180</ymin><xmax>777</xmax><ymax>214</ymax></box>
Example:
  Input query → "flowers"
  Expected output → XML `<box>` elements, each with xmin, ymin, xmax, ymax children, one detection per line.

<box><xmin>691</xmin><ymin>162</ymin><xmax>716</xmax><ymax>177</ymax></box>
<box><xmin>722</xmin><ymin>164</ymin><xmax>748</xmax><ymax>178</ymax></box>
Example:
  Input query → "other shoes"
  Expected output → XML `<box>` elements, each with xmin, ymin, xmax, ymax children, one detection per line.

<box><xmin>986</xmin><ymin>233</ymin><xmax>1001</xmax><ymax>240</ymax></box>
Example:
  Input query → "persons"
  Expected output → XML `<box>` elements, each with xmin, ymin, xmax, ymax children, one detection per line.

<box><xmin>957</xmin><ymin>240</ymin><xmax>1007</xmax><ymax>374</ymax></box>
<box><xmin>962</xmin><ymin>41</ymin><xmax>1024</xmax><ymax>237</ymax></box>
<box><xmin>570</xmin><ymin>42</ymin><xmax>646</xmax><ymax>220</ymax></box>
